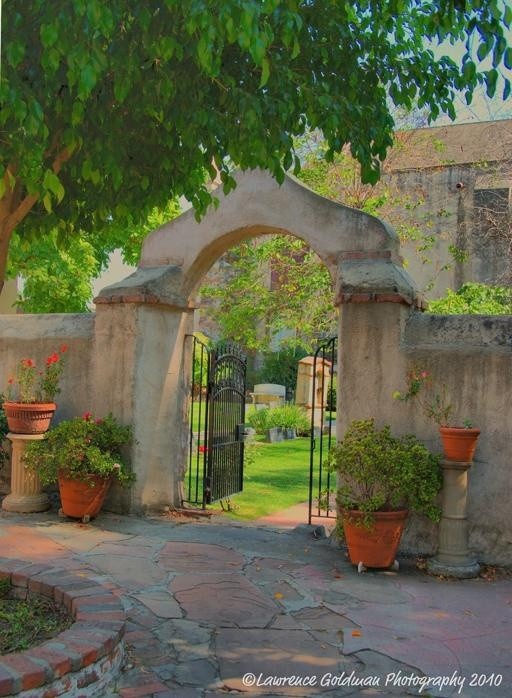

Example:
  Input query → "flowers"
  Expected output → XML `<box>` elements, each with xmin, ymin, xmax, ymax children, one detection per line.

<box><xmin>24</xmin><ymin>412</ymin><xmax>146</xmax><ymax>488</ymax></box>
<box><xmin>393</xmin><ymin>354</ymin><xmax>481</xmax><ymax>426</ymax></box>
<box><xmin>2</xmin><ymin>335</ymin><xmax>70</xmax><ymax>401</ymax></box>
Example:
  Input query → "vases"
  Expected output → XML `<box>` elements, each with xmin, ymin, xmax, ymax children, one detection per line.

<box><xmin>1</xmin><ymin>402</ymin><xmax>56</xmax><ymax>432</ymax></box>
<box><xmin>56</xmin><ymin>471</ymin><xmax>117</xmax><ymax>516</ymax></box>
<box><xmin>439</xmin><ymin>427</ymin><xmax>487</xmax><ymax>460</ymax></box>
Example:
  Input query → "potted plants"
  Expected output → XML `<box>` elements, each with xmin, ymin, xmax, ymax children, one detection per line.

<box><xmin>312</xmin><ymin>417</ymin><xmax>442</xmax><ymax>568</ymax></box>
